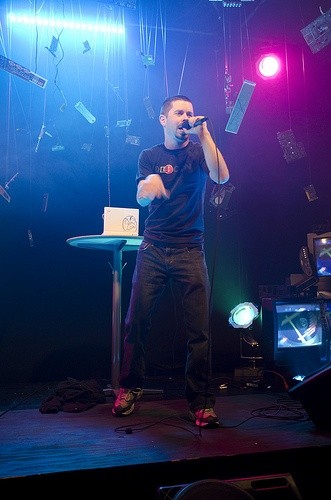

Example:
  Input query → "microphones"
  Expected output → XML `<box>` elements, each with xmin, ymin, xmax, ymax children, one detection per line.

<box><xmin>182</xmin><ymin>117</ymin><xmax>208</xmax><ymax>130</ymax></box>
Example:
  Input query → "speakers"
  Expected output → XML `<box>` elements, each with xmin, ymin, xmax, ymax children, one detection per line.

<box><xmin>288</xmin><ymin>363</ymin><xmax>331</xmax><ymax>432</ymax></box>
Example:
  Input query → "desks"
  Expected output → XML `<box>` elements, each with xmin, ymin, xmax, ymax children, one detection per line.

<box><xmin>66</xmin><ymin>235</ymin><xmax>147</xmax><ymax>406</ymax></box>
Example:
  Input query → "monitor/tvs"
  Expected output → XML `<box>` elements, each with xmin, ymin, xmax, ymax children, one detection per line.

<box><xmin>272</xmin><ymin>302</ymin><xmax>326</xmax><ymax>351</ymax></box>
<box><xmin>306</xmin><ymin>232</ymin><xmax>331</xmax><ymax>282</ymax></box>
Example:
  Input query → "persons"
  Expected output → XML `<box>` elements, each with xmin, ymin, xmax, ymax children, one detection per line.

<box><xmin>112</xmin><ymin>95</ymin><xmax>229</xmax><ymax>426</ymax></box>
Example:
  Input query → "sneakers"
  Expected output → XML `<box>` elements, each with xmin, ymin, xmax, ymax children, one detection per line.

<box><xmin>190</xmin><ymin>406</ymin><xmax>220</xmax><ymax>428</ymax></box>
<box><xmin>112</xmin><ymin>386</ymin><xmax>144</xmax><ymax>416</ymax></box>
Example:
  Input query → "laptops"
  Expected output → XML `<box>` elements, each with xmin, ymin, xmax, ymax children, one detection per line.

<box><xmin>101</xmin><ymin>207</ymin><xmax>139</xmax><ymax>236</ymax></box>
